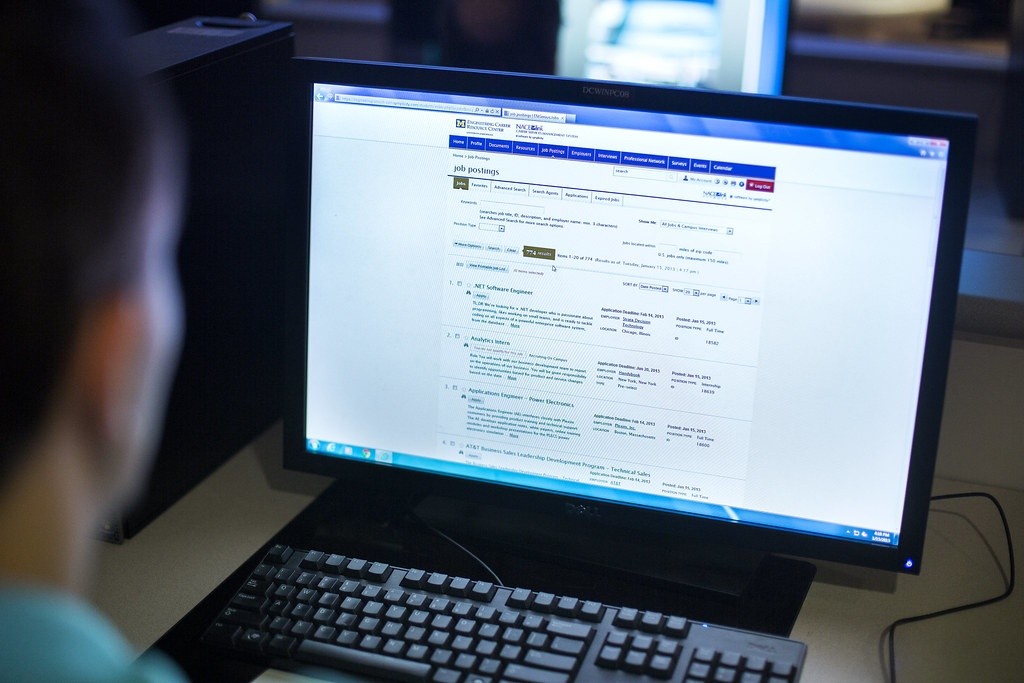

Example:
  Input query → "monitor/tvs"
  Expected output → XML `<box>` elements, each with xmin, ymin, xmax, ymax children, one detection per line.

<box><xmin>543</xmin><ymin>0</ymin><xmax>794</xmax><ymax>95</ymax></box>
<box><xmin>280</xmin><ymin>56</ymin><xmax>975</xmax><ymax>600</ymax></box>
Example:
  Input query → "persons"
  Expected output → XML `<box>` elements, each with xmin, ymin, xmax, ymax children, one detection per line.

<box><xmin>0</xmin><ymin>0</ymin><xmax>190</xmax><ymax>683</ymax></box>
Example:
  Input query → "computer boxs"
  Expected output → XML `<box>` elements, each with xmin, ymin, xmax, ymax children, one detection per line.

<box><xmin>89</xmin><ymin>14</ymin><xmax>297</xmax><ymax>543</ymax></box>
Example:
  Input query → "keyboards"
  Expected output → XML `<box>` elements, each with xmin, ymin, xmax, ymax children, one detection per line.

<box><xmin>205</xmin><ymin>542</ymin><xmax>809</xmax><ymax>683</ymax></box>
